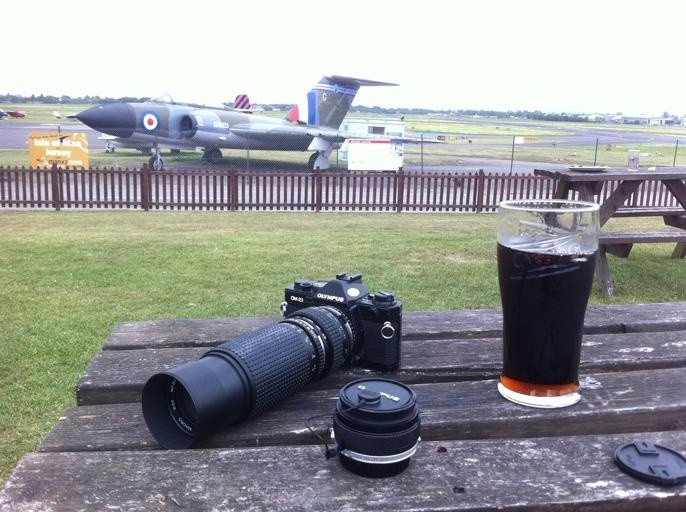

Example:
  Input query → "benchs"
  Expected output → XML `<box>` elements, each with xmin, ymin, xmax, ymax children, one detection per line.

<box><xmin>598</xmin><ymin>231</ymin><xmax>686</xmax><ymax>259</ymax></box>
<box><xmin>612</xmin><ymin>205</ymin><xmax>686</xmax><ymax>217</ymax></box>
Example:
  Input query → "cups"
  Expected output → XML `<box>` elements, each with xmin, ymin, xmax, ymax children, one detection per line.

<box><xmin>627</xmin><ymin>150</ymin><xmax>640</xmax><ymax>171</ymax></box>
<box><xmin>494</xmin><ymin>199</ymin><xmax>600</xmax><ymax>411</ymax></box>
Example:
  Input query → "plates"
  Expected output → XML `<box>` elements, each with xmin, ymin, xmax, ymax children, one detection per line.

<box><xmin>569</xmin><ymin>165</ymin><xmax>608</xmax><ymax>172</ymax></box>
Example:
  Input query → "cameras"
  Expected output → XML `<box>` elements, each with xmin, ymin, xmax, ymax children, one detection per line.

<box><xmin>142</xmin><ymin>272</ymin><xmax>402</xmax><ymax>450</ymax></box>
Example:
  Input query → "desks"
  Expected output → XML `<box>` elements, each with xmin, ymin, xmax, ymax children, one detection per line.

<box><xmin>533</xmin><ymin>165</ymin><xmax>686</xmax><ymax>297</ymax></box>
<box><xmin>0</xmin><ymin>301</ymin><xmax>686</xmax><ymax>512</ymax></box>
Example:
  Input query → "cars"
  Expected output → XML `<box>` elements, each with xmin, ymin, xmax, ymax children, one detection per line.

<box><xmin>0</xmin><ymin>109</ymin><xmax>27</xmax><ymax>119</ymax></box>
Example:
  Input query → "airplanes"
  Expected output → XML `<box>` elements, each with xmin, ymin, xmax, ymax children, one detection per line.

<box><xmin>76</xmin><ymin>74</ymin><xmax>449</xmax><ymax>172</ymax></box>
